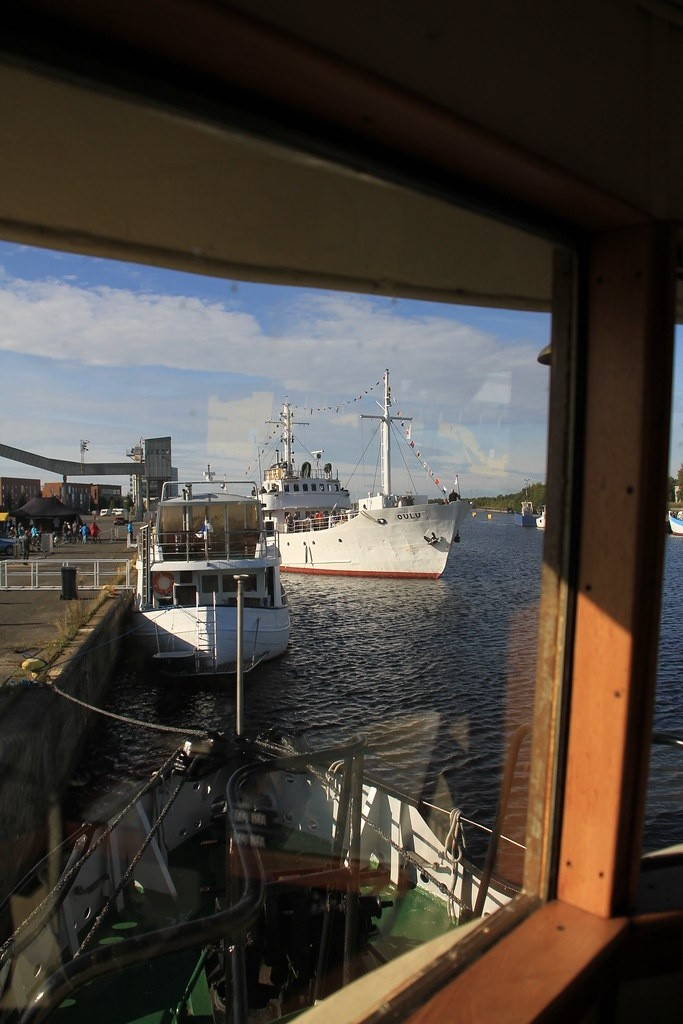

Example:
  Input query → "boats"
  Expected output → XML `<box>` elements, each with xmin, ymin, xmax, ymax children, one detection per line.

<box><xmin>668</xmin><ymin>510</ymin><xmax>683</xmax><ymax>535</ymax></box>
<box><xmin>130</xmin><ymin>463</ymin><xmax>295</xmax><ymax>680</ymax></box>
<box><xmin>256</xmin><ymin>368</ymin><xmax>474</xmax><ymax>580</ymax></box>
<box><xmin>512</xmin><ymin>478</ymin><xmax>546</xmax><ymax>528</ymax></box>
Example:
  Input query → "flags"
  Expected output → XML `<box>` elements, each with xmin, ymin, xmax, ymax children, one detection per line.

<box><xmin>201</xmin><ymin>520</ymin><xmax>213</xmax><ymax>534</ymax></box>
<box><xmin>388</xmin><ymin>389</ymin><xmax>449</xmax><ymax>495</ymax></box>
<box><xmin>289</xmin><ymin>377</ymin><xmax>385</xmax><ymax>417</ymax></box>
<box><xmin>243</xmin><ymin>415</ymin><xmax>282</xmax><ymax>474</ymax></box>
<box><xmin>454</xmin><ymin>476</ymin><xmax>458</xmax><ymax>485</ymax></box>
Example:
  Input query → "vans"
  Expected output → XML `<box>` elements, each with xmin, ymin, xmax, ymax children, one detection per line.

<box><xmin>100</xmin><ymin>508</ymin><xmax>128</xmax><ymax>526</ymax></box>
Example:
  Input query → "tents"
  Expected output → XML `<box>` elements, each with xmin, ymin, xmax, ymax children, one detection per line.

<box><xmin>8</xmin><ymin>496</ymin><xmax>79</xmax><ymax>517</ymax></box>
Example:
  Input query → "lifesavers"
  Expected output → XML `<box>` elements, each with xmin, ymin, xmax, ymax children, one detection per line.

<box><xmin>152</xmin><ymin>572</ymin><xmax>175</xmax><ymax>595</ymax></box>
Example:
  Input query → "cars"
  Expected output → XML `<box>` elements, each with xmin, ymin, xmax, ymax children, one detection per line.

<box><xmin>0</xmin><ymin>538</ymin><xmax>17</xmax><ymax>555</ymax></box>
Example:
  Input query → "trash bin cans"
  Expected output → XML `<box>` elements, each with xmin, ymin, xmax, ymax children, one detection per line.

<box><xmin>59</xmin><ymin>566</ymin><xmax>80</xmax><ymax>600</ymax></box>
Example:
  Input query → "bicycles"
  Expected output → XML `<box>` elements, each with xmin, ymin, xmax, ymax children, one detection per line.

<box><xmin>62</xmin><ymin>531</ymin><xmax>104</xmax><ymax>545</ymax></box>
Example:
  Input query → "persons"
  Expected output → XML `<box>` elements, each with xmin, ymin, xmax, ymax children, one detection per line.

<box><xmin>90</xmin><ymin>522</ymin><xmax>101</xmax><ymax>543</ymax></box>
<box><xmin>250</xmin><ymin>459</ymin><xmax>328</xmax><ymax>497</ymax></box>
<box><xmin>91</xmin><ymin>509</ymin><xmax>96</xmax><ymax>520</ymax></box>
<box><xmin>79</xmin><ymin>523</ymin><xmax>90</xmax><ymax>543</ymax></box>
<box><xmin>22</xmin><ymin>530</ymin><xmax>30</xmax><ymax>565</ymax></box>
<box><xmin>127</xmin><ymin>520</ymin><xmax>133</xmax><ymax>541</ymax></box>
<box><xmin>7</xmin><ymin>514</ymin><xmax>82</xmax><ymax>547</ymax></box>
<box><xmin>286</xmin><ymin>511</ymin><xmax>346</xmax><ymax>533</ymax></box>
